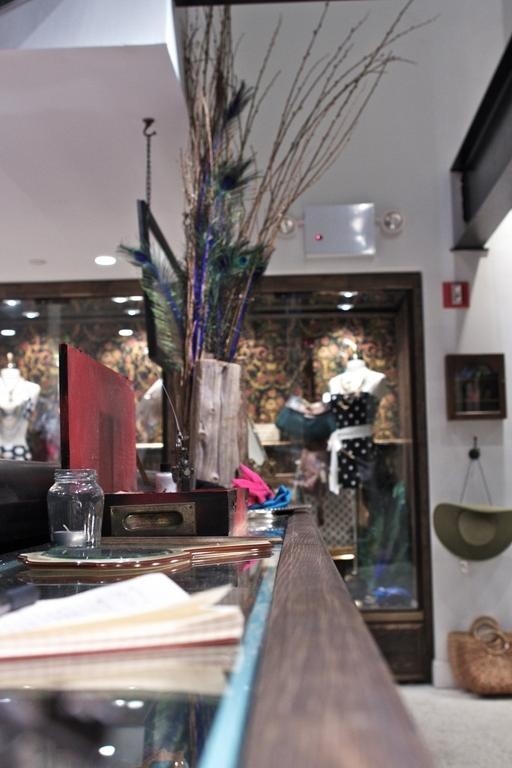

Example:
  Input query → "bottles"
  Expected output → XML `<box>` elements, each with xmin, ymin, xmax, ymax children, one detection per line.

<box><xmin>47</xmin><ymin>468</ymin><xmax>104</xmax><ymax>549</ymax></box>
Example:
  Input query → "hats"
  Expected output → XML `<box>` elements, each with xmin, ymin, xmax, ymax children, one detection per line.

<box><xmin>435</xmin><ymin>503</ymin><xmax>512</xmax><ymax>561</ymax></box>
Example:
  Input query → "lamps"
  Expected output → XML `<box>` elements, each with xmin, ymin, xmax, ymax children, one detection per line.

<box><xmin>273</xmin><ymin>201</ymin><xmax>406</xmax><ymax>258</ymax></box>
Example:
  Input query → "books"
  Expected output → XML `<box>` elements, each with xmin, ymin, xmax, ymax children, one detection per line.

<box><xmin>184</xmin><ymin>538</ymin><xmax>271</xmax><ymax>562</ymax></box>
<box><xmin>0</xmin><ymin>656</ymin><xmax>235</xmax><ymax>703</ymax></box>
<box><xmin>1</xmin><ymin>570</ymin><xmax>244</xmax><ymax>661</ymax></box>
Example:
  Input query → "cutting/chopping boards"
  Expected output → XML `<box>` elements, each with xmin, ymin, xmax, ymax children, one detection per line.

<box><xmin>17</xmin><ymin>537</ymin><xmax>272</xmax><ymax>572</ymax></box>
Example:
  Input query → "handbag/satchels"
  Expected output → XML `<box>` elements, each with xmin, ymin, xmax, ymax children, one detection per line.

<box><xmin>447</xmin><ymin>617</ymin><xmax>511</xmax><ymax>699</ymax></box>
<box><xmin>276</xmin><ymin>395</ymin><xmax>335</xmax><ymax>437</ymax></box>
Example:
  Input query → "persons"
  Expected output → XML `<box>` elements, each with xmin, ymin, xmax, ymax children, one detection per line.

<box><xmin>322</xmin><ymin>352</ymin><xmax>389</xmax><ymax>493</ymax></box>
<box><xmin>0</xmin><ymin>367</ymin><xmax>41</xmax><ymax>462</ymax></box>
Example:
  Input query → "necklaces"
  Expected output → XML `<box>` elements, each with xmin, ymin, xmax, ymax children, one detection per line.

<box><xmin>343</xmin><ymin>379</ymin><xmax>365</xmax><ymax>394</ymax></box>
<box><xmin>9</xmin><ymin>389</ymin><xmax>16</xmax><ymax>401</ymax></box>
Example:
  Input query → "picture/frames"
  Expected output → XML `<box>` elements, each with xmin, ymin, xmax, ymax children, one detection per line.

<box><xmin>444</xmin><ymin>352</ymin><xmax>507</xmax><ymax>421</ymax></box>
